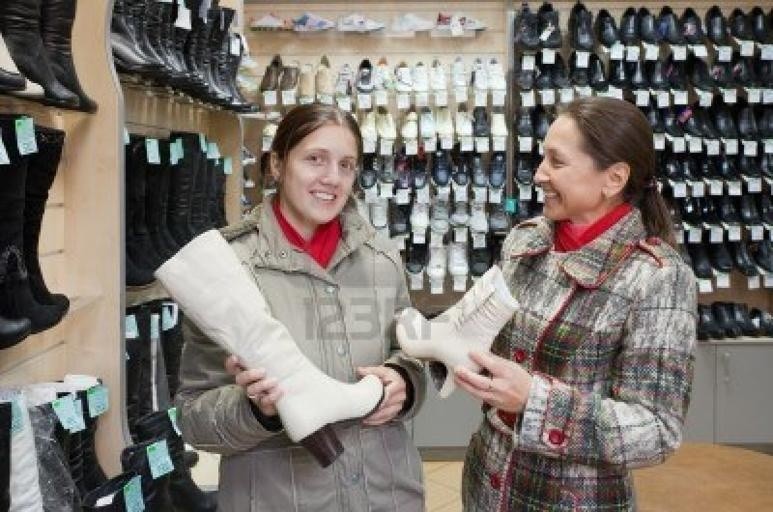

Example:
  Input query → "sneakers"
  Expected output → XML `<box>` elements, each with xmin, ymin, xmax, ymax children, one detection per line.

<box><xmin>259</xmin><ymin>109</ymin><xmax>286</xmax><ymax>146</ymax></box>
<box><xmin>355</xmin><ymin>147</ymin><xmax>397</xmax><ymax>193</ymax></box>
<box><xmin>429</xmin><ymin>56</ymin><xmax>446</xmax><ymax>91</ymax></box>
<box><xmin>386</xmin><ymin>13</ymin><xmax>439</xmax><ymax>33</ymax></box>
<box><xmin>433</xmin><ymin>103</ymin><xmax>456</xmax><ymax>137</ymax></box>
<box><xmin>333</xmin><ymin>60</ymin><xmax>356</xmax><ymax>97</ymax></box>
<box><xmin>447</xmin><ymin>142</ymin><xmax>488</xmax><ymax>187</ymax></box>
<box><xmin>456</xmin><ymin>100</ymin><xmax>471</xmax><ymax>140</ymax></box>
<box><xmin>434</xmin><ymin>11</ymin><xmax>489</xmax><ymax>34</ymax></box>
<box><xmin>361</xmin><ymin>196</ymin><xmax>408</xmax><ymax>238</ymax></box>
<box><xmin>297</xmin><ymin>53</ymin><xmax>335</xmax><ymax>101</ymax></box>
<box><xmin>287</xmin><ymin>12</ymin><xmax>337</xmax><ymax>34</ymax></box>
<box><xmin>410</xmin><ymin>196</ymin><xmax>453</xmax><ymax>238</ymax></box>
<box><xmin>356</xmin><ymin>53</ymin><xmax>392</xmax><ymax>94</ymax></box>
<box><xmin>488</xmin><ymin>195</ymin><xmax>512</xmax><ymax>234</ymax></box>
<box><xmin>470</xmin><ymin>103</ymin><xmax>510</xmax><ymax>138</ymax></box>
<box><xmin>444</xmin><ymin>194</ymin><xmax>470</xmax><ymax>231</ymax></box>
<box><xmin>469</xmin><ymin>56</ymin><xmax>507</xmax><ymax>92</ymax></box>
<box><xmin>448</xmin><ymin>55</ymin><xmax>469</xmax><ymax>89</ymax></box>
<box><xmin>430</xmin><ymin>143</ymin><xmax>450</xmax><ymax>188</ymax></box>
<box><xmin>248</xmin><ymin>10</ymin><xmax>291</xmax><ymax>31</ymax></box>
<box><xmin>334</xmin><ymin>10</ymin><xmax>385</xmax><ymax>33</ymax></box>
<box><xmin>396</xmin><ymin>236</ymin><xmax>503</xmax><ymax>282</ymax></box>
<box><xmin>346</xmin><ymin>103</ymin><xmax>400</xmax><ymax>144</ymax></box>
<box><xmin>400</xmin><ymin>104</ymin><xmax>434</xmax><ymax>140</ymax></box>
<box><xmin>392</xmin><ymin>60</ymin><xmax>427</xmax><ymax>93</ymax></box>
<box><xmin>395</xmin><ymin>147</ymin><xmax>427</xmax><ymax>194</ymax></box>
<box><xmin>466</xmin><ymin>191</ymin><xmax>489</xmax><ymax>238</ymax></box>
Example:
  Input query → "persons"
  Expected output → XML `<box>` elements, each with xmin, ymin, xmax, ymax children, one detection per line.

<box><xmin>423</xmin><ymin>97</ymin><xmax>699</xmax><ymax>512</ymax></box>
<box><xmin>175</xmin><ymin>102</ymin><xmax>432</xmax><ymax>511</ymax></box>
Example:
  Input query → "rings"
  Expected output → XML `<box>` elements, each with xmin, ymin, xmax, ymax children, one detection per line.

<box><xmin>485</xmin><ymin>380</ymin><xmax>492</xmax><ymax>392</ymax></box>
<box><xmin>247</xmin><ymin>393</ymin><xmax>257</xmax><ymax>399</ymax></box>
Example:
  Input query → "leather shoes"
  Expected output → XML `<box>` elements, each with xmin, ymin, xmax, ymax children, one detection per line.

<box><xmin>692</xmin><ymin>298</ymin><xmax>772</xmax><ymax>344</ymax></box>
<box><xmin>513</xmin><ymin>50</ymin><xmax>772</xmax><ymax>95</ymax></box>
<box><xmin>618</xmin><ymin>3</ymin><xmax>772</xmax><ymax>47</ymax></box>
<box><xmin>642</xmin><ymin>97</ymin><xmax>771</xmax><ymax>142</ymax></box>
<box><xmin>566</xmin><ymin>1</ymin><xmax>597</xmax><ymax>50</ymax></box>
<box><xmin>487</xmin><ymin>147</ymin><xmax>508</xmax><ymax>190</ymax></box>
<box><xmin>513</xmin><ymin>103</ymin><xmax>557</xmax><ymax>142</ymax></box>
<box><xmin>594</xmin><ymin>6</ymin><xmax>622</xmax><ymax>50</ymax></box>
<box><xmin>682</xmin><ymin>232</ymin><xmax>773</xmax><ymax>280</ymax></box>
<box><xmin>661</xmin><ymin>188</ymin><xmax>773</xmax><ymax>230</ymax></box>
<box><xmin>653</xmin><ymin>147</ymin><xmax>772</xmax><ymax>184</ymax></box>
<box><xmin>514</xmin><ymin>0</ymin><xmax>567</xmax><ymax>50</ymax></box>
<box><xmin>259</xmin><ymin>49</ymin><xmax>299</xmax><ymax>97</ymax></box>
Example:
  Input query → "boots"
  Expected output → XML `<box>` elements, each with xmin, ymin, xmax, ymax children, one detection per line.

<box><xmin>149</xmin><ymin>225</ymin><xmax>385</xmax><ymax>471</ymax></box>
<box><xmin>106</xmin><ymin>0</ymin><xmax>263</xmax><ymax>117</ymax></box>
<box><xmin>1</xmin><ymin>0</ymin><xmax>102</xmax><ymax>114</ymax></box>
<box><xmin>0</xmin><ymin>112</ymin><xmax>73</xmax><ymax>353</ymax></box>
<box><xmin>392</xmin><ymin>261</ymin><xmax>524</xmax><ymax>401</ymax></box>
<box><xmin>122</xmin><ymin>128</ymin><xmax>232</xmax><ymax>292</ymax></box>
<box><xmin>122</xmin><ymin>296</ymin><xmax>186</xmax><ymax>447</ymax></box>
<box><xmin>0</xmin><ymin>374</ymin><xmax>219</xmax><ymax>511</ymax></box>
